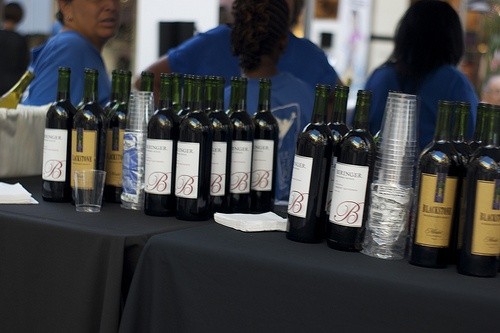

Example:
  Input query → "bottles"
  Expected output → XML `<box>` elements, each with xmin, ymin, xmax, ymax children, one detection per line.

<box><xmin>320</xmin><ymin>84</ymin><xmax>350</xmax><ymax>242</ymax></box>
<box><xmin>323</xmin><ymin>90</ymin><xmax>376</xmax><ymax>254</ymax></box>
<box><xmin>141</xmin><ymin>72</ymin><xmax>280</xmax><ymax>220</ymax></box>
<box><xmin>284</xmin><ymin>83</ymin><xmax>334</xmax><ymax>245</ymax></box>
<box><xmin>0</xmin><ymin>71</ymin><xmax>35</xmax><ymax>109</ymax></box>
<box><xmin>67</xmin><ymin>68</ymin><xmax>131</xmax><ymax>205</ymax></box>
<box><xmin>407</xmin><ymin>101</ymin><xmax>500</xmax><ymax>279</ymax></box>
<box><xmin>41</xmin><ymin>67</ymin><xmax>75</xmax><ymax>203</ymax></box>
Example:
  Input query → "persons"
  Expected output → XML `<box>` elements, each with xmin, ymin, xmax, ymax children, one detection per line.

<box><xmin>0</xmin><ymin>3</ymin><xmax>29</xmax><ymax>98</ymax></box>
<box><xmin>351</xmin><ymin>0</ymin><xmax>481</xmax><ymax>151</ymax></box>
<box><xmin>132</xmin><ymin>1</ymin><xmax>348</xmax><ymax>203</ymax></box>
<box><xmin>17</xmin><ymin>0</ymin><xmax>123</xmax><ymax>106</ymax></box>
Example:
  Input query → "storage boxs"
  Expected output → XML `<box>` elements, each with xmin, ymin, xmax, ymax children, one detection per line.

<box><xmin>0</xmin><ymin>104</ymin><xmax>48</xmax><ymax>177</ymax></box>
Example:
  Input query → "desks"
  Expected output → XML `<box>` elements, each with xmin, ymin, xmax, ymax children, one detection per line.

<box><xmin>0</xmin><ymin>170</ymin><xmax>500</xmax><ymax>333</ymax></box>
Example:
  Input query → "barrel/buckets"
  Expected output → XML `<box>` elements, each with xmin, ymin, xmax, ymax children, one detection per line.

<box><xmin>0</xmin><ymin>109</ymin><xmax>48</xmax><ymax>179</ymax></box>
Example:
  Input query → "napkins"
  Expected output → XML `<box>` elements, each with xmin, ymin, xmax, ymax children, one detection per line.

<box><xmin>0</xmin><ymin>182</ymin><xmax>40</xmax><ymax>205</ymax></box>
<box><xmin>214</xmin><ymin>211</ymin><xmax>288</xmax><ymax>233</ymax></box>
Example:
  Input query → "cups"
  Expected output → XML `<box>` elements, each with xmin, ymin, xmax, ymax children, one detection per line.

<box><xmin>360</xmin><ymin>91</ymin><xmax>418</xmax><ymax>262</ymax></box>
<box><xmin>73</xmin><ymin>169</ymin><xmax>106</xmax><ymax>212</ymax></box>
<box><xmin>119</xmin><ymin>91</ymin><xmax>153</xmax><ymax>211</ymax></box>
